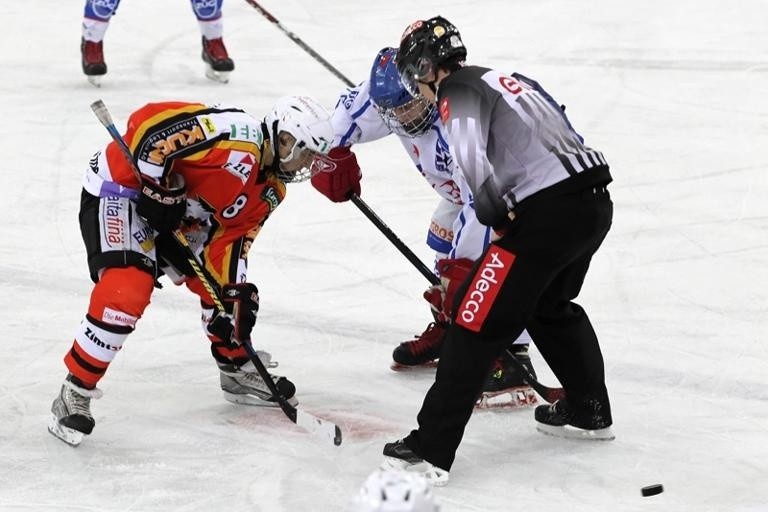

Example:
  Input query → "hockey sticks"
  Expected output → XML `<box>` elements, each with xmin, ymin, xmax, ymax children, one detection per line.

<box><xmin>348</xmin><ymin>192</ymin><xmax>565</xmax><ymax>402</ymax></box>
<box><xmin>91</xmin><ymin>99</ymin><xmax>342</xmax><ymax>448</ymax></box>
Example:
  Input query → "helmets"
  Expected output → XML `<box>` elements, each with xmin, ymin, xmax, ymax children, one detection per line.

<box><xmin>265</xmin><ymin>16</ymin><xmax>467</xmax><ymax>183</ymax></box>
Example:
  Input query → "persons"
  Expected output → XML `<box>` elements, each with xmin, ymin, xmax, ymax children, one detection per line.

<box><xmin>346</xmin><ymin>469</ymin><xmax>450</xmax><ymax>512</ymax></box>
<box><xmin>317</xmin><ymin>46</ymin><xmax>539</xmax><ymax>410</ymax></box>
<box><xmin>382</xmin><ymin>15</ymin><xmax>618</xmax><ymax>487</ymax></box>
<box><xmin>79</xmin><ymin>0</ymin><xmax>238</xmax><ymax>77</ymax></box>
<box><xmin>50</xmin><ymin>97</ymin><xmax>328</xmax><ymax>436</ymax></box>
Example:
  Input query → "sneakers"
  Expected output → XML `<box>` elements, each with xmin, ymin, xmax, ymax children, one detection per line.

<box><xmin>210</xmin><ymin>344</ymin><xmax>299</xmax><ymax>407</ymax></box>
<box><xmin>391</xmin><ymin>321</ymin><xmax>446</xmax><ymax>368</ymax></box>
<box><xmin>383</xmin><ymin>435</ymin><xmax>451</xmax><ymax>486</ymax></box>
<box><xmin>535</xmin><ymin>397</ymin><xmax>615</xmax><ymax>440</ymax></box>
<box><xmin>202</xmin><ymin>36</ymin><xmax>234</xmax><ymax>71</ymax></box>
<box><xmin>81</xmin><ymin>38</ymin><xmax>106</xmax><ymax>75</ymax></box>
<box><xmin>474</xmin><ymin>344</ymin><xmax>538</xmax><ymax>410</ymax></box>
<box><xmin>47</xmin><ymin>373</ymin><xmax>102</xmax><ymax>448</ymax></box>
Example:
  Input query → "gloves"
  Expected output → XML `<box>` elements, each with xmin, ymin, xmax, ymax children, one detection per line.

<box><xmin>136</xmin><ymin>174</ymin><xmax>188</xmax><ymax>234</ymax></box>
<box><xmin>208</xmin><ymin>282</ymin><xmax>258</xmax><ymax>350</ymax></box>
<box><xmin>424</xmin><ymin>258</ymin><xmax>478</xmax><ymax>324</ymax></box>
<box><xmin>310</xmin><ymin>150</ymin><xmax>363</xmax><ymax>201</ymax></box>
